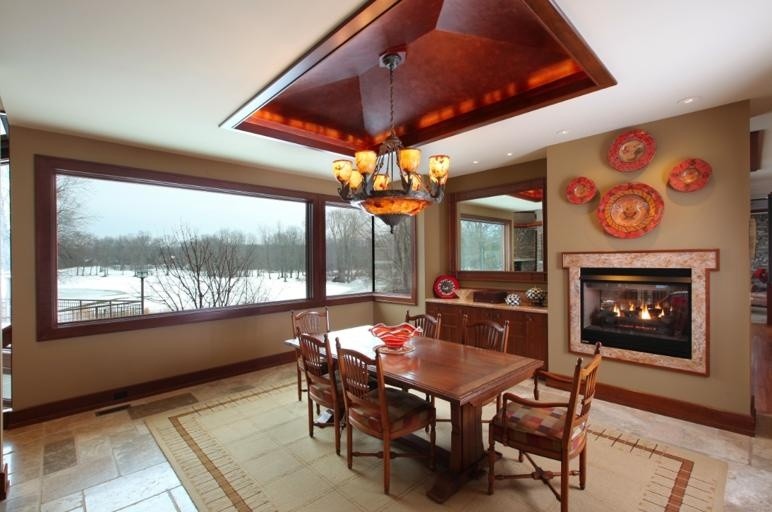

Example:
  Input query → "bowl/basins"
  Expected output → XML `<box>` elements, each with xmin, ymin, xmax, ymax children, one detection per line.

<box><xmin>368</xmin><ymin>322</ymin><xmax>424</xmax><ymax>349</ymax></box>
<box><xmin>452</xmin><ymin>289</ymin><xmax>472</xmax><ymax>300</ymax></box>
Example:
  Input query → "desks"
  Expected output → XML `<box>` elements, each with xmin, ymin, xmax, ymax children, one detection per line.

<box><xmin>285</xmin><ymin>323</ymin><xmax>545</xmax><ymax>503</ymax></box>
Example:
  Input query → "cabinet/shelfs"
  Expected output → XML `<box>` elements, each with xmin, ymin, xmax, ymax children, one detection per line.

<box><xmin>427</xmin><ymin>302</ymin><xmax>548</xmax><ymax>363</ymax></box>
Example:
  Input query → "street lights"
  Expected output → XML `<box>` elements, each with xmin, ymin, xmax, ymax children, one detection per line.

<box><xmin>136</xmin><ymin>270</ymin><xmax>148</xmax><ymax>315</ymax></box>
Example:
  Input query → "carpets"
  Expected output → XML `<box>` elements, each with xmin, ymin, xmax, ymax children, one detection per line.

<box><xmin>141</xmin><ymin>378</ymin><xmax>731</xmax><ymax>512</ymax></box>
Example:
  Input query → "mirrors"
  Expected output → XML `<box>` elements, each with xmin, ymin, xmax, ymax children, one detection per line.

<box><xmin>445</xmin><ymin>179</ymin><xmax>546</xmax><ymax>284</ymax></box>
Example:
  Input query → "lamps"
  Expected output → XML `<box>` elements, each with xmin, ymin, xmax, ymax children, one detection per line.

<box><xmin>332</xmin><ymin>46</ymin><xmax>452</xmax><ymax>232</ymax></box>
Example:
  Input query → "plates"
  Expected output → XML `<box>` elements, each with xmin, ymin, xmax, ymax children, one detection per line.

<box><xmin>372</xmin><ymin>344</ymin><xmax>416</xmax><ymax>355</ymax></box>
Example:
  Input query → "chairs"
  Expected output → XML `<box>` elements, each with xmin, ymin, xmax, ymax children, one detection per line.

<box><xmin>297</xmin><ymin>327</ymin><xmax>345</xmax><ymax>456</ymax></box>
<box><xmin>489</xmin><ymin>343</ymin><xmax>601</xmax><ymax>509</ymax></box>
<box><xmin>336</xmin><ymin>337</ymin><xmax>437</xmax><ymax>494</ymax></box>
<box><xmin>405</xmin><ymin>305</ymin><xmax>509</xmax><ymax>433</ymax></box>
<box><xmin>293</xmin><ymin>308</ymin><xmax>329</xmax><ymax>401</ymax></box>
